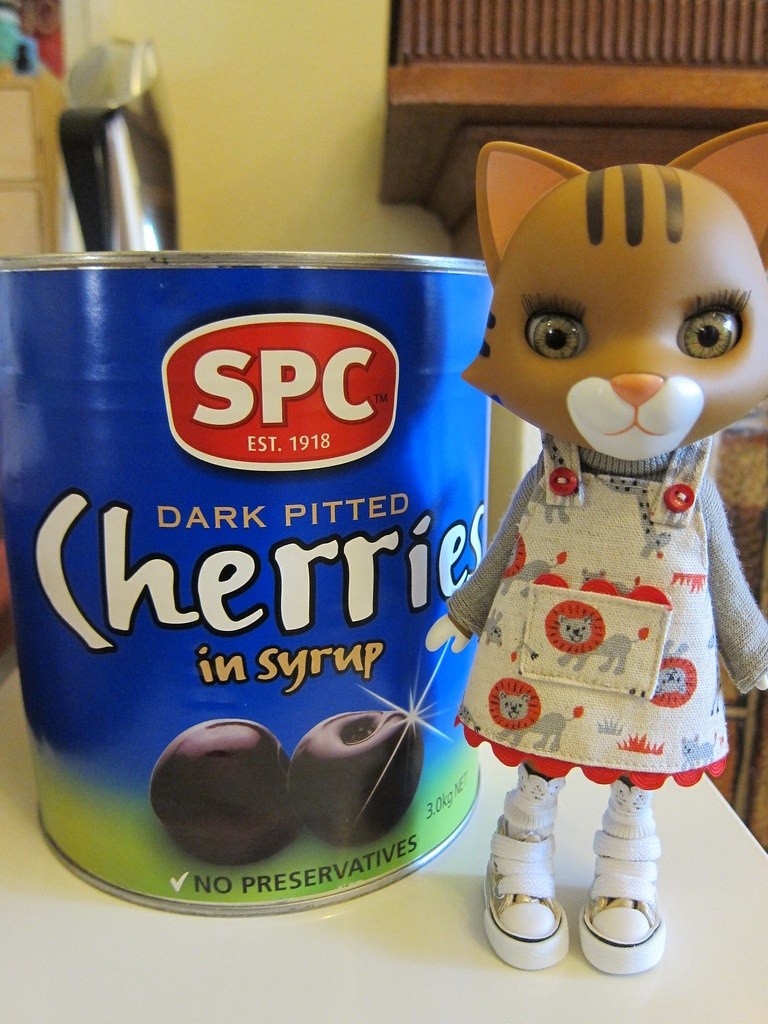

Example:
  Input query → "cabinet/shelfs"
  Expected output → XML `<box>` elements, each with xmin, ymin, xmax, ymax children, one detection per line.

<box><xmin>0</xmin><ymin>70</ymin><xmax>65</xmax><ymax>254</ymax></box>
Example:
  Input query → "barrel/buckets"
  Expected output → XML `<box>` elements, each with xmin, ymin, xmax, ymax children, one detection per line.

<box><xmin>0</xmin><ymin>252</ymin><xmax>493</xmax><ymax>919</ymax></box>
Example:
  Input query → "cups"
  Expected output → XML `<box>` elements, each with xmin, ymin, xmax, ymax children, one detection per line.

<box><xmin>0</xmin><ymin>9</ymin><xmax>38</xmax><ymax>73</ymax></box>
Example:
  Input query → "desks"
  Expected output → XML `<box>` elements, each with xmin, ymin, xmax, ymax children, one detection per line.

<box><xmin>0</xmin><ymin>653</ymin><xmax>768</xmax><ymax>1024</ymax></box>
